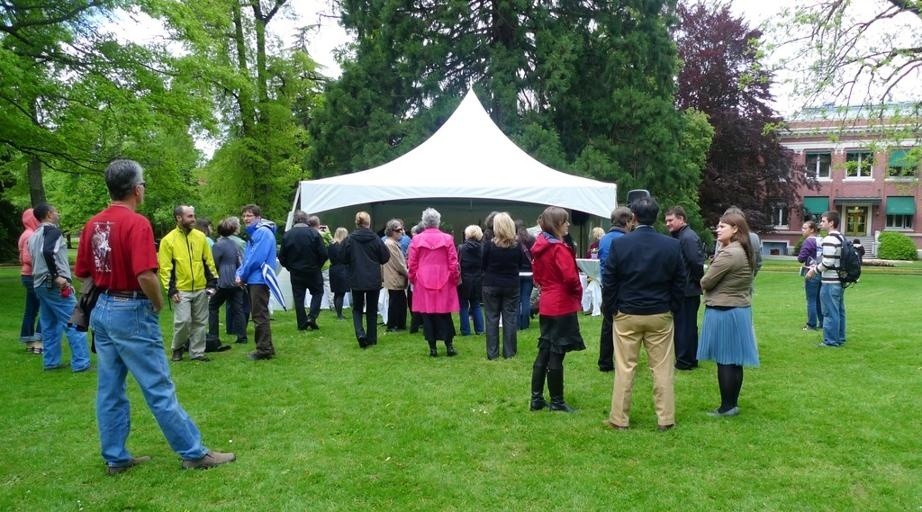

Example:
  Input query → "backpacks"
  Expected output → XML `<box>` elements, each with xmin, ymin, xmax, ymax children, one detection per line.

<box><xmin>832</xmin><ymin>233</ymin><xmax>861</xmax><ymax>289</ymax></box>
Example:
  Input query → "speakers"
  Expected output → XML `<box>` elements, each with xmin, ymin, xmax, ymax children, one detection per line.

<box><xmin>626</xmin><ymin>189</ymin><xmax>652</xmax><ymax>207</ymax></box>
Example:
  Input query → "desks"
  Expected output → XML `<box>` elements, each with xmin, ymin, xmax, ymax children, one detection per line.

<box><xmin>575</xmin><ymin>257</ymin><xmax>603</xmax><ymax>315</ymax></box>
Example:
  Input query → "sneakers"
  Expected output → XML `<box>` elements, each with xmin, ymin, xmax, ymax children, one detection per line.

<box><xmin>172</xmin><ymin>338</ymin><xmax>248</xmax><ymax>361</ymax></box>
<box><xmin>106</xmin><ymin>456</ymin><xmax>150</xmax><ymax>474</ymax></box>
<box><xmin>182</xmin><ymin>451</ymin><xmax>236</xmax><ymax>468</ymax></box>
<box><xmin>250</xmin><ymin>353</ymin><xmax>272</xmax><ymax>360</ymax></box>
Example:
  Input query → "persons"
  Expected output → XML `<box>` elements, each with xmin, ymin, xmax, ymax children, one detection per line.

<box><xmin>693</xmin><ymin>210</ymin><xmax>762</xmax><ymax>418</ymax></box>
<box><xmin>528</xmin><ymin>205</ymin><xmax>587</xmax><ymax>414</ymax></box>
<box><xmin>17</xmin><ymin>209</ymin><xmax>42</xmax><ymax>345</ymax></box>
<box><xmin>25</xmin><ymin>202</ymin><xmax>91</xmax><ymax>374</ymax></box>
<box><xmin>599</xmin><ymin>196</ymin><xmax>688</xmax><ymax>431</ymax></box>
<box><xmin>75</xmin><ymin>158</ymin><xmax>236</xmax><ymax>475</ymax></box>
<box><xmin>406</xmin><ymin>206</ymin><xmax>708</xmax><ymax>372</ymax></box>
<box><xmin>157</xmin><ymin>203</ymin><xmax>276</xmax><ymax>361</ymax></box>
<box><xmin>714</xmin><ymin>205</ymin><xmax>762</xmax><ymax>278</ymax></box>
<box><xmin>278</xmin><ymin>209</ymin><xmax>413</xmax><ymax>349</ymax></box>
<box><xmin>804</xmin><ymin>211</ymin><xmax>845</xmax><ymax>348</ymax></box>
<box><xmin>797</xmin><ymin>219</ymin><xmax>825</xmax><ymax>331</ymax></box>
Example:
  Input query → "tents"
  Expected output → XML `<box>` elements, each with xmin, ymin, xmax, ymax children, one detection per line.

<box><xmin>269</xmin><ymin>74</ymin><xmax>617</xmax><ymax>310</ymax></box>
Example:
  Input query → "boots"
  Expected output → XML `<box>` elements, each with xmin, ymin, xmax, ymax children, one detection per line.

<box><xmin>530</xmin><ymin>365</ymin><xmax>550</xmax><ymax>410</ymax></box>
<box><xmin>547</xmin><ymin>366</ymin><xmax>575</xmax><ymax>412</ymax></box>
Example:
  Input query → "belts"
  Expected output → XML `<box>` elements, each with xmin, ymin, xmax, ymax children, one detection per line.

<box><xmin>103</xmin><ymin>289</ymin><xmax>146</xmax><ymax>300</ymax></box>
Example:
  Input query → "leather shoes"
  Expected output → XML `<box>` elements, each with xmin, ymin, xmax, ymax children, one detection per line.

<box><xmin>447</xmin><ymin>350</ymin><xmax>457</xmax><ymax>356</ymax></box>
<box><xmin>307</xmin><ymin>317</ymin><xmax>319</xmax><ymax>330</ymax></box>
<box><xmin>706</xmin><ymin>408</ymin><xmax>736</xmax><ymax>416</ymax></box>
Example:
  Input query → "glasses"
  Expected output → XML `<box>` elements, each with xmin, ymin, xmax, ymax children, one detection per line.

<box><xmin>393</xmin><ymin>229</ymin><xmax>402</xmax><ymax>233</ymax></box>
